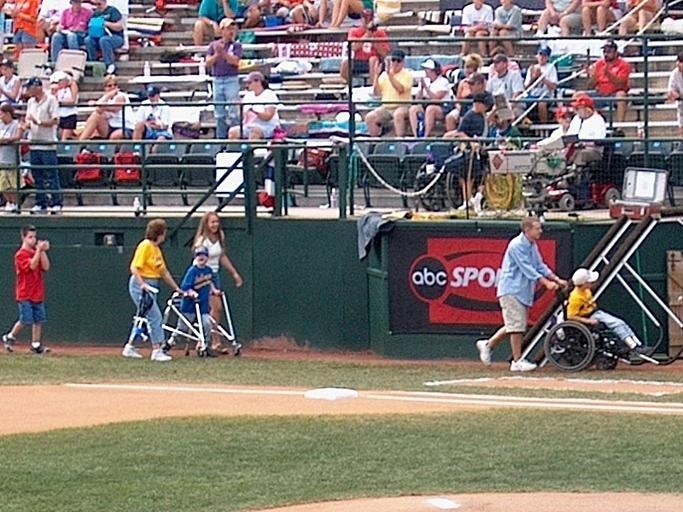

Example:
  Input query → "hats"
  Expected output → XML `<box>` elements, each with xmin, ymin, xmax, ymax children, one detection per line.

<box><xmin>463</xmin><ymin>54</ymin><xmax>507</xmax><ymax>84</ymax></box>
<box><xmin>195</xmin><ymin>247</ymin><xmax>208</xmax><ymax>256</ymax></box>
<box><xmin>537</xmin><ymin>45</ymin><xmax>550</xmax><ymax>55</ymax></box>
<box><xmin>421</xmin><ymin>59</ymin><xmax>439</xmax><ymax>69</ymax></box>
<box><xmin>219</xmin><ymin>18</ymin><xmax>235</xmax><ymax>29</ymax></box>
<box><xmin>391</xmin><ymin>50</ymin><xmax>405</xmax><ymax>60</ymax></box>
<box><xmin>0</xmin><ymin>60</ymin><xmax>13</xmax><ymax>67</ymax></box>
<box><xmin>243</xmin><ymin>72</ymin><xmax>264</xmax><ymax>82</ymax></box>
<box><xmin>557</xmin><ymin>96</ymin><xmax>594</xmax><ymax>118</ymax></box>
<box><xmin>22</xmin><ymin>77</ymin><xmax>42</xmax><ymax>87</ymax></box>
<box><xmin>571</xmin><ymin>268</ymin><xmax>599</xmax><ymax>286</ymax></box>
<box><xmin>601</xmin><ymin>41</ymin><xmax>617</xmax><ymax>48</ymax></box>
<box><xmin>146</xmin><ymin>86</ymin><xmax>159</xmax><ymax>96</ymax></box>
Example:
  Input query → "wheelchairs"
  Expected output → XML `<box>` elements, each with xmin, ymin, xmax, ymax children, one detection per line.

<box><xmin>414</xmin><ymin>143</ymin><xmax>477</xmax><ymax>211</ymax></box>
<box><xmin>535</xmin><ymin>282</ymin><xmax>676</xmax><ymax>371</ymax></box>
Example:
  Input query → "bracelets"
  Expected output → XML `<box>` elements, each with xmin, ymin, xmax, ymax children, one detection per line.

<box><xmin>554</xmin><ymin>277</ymin><xmax>559</xmax><ymax>283</ymax></box>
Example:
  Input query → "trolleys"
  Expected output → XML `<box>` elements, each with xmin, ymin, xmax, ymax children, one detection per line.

<box><xmin>155</xmin><ymin>286</ymin><xmax>252</xmax><ymax>359</ymax></box>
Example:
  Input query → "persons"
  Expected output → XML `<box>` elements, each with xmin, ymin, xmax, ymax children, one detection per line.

<box><xmin>476</xmin><ymin>215</ymin><xmax>568</xmax><ymax>373</ymax></box>
<box><xmin>161</xmin><ymin>246</ymin><xmax>221</xmax><ymax>357</ymax></box>
<box><xmin>1</xmin><ymin>224</ymin><xmax>51</xmax><ymax>356</ymax></box>
<box><xmin>122</xmin><ymin>218</ymin><xmax>187</xmax><ymax>360</ymax></box>
<box><xmin>1</xmin><ymin>0</ymin><xmax>682</xmax><ymax>218</ymax></box>
<box><xmin>566</xmin><ymin>267</ymin><xmax>656</xmax><ymax>361</ymax></box>
<box><xmin>192</xmin><ymin>211</ymin><xmax>245</xmax><ymax>356</ymax></box>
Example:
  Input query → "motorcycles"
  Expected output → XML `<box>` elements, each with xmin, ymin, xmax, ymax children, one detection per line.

<box><xmin>519</xmin><ymin>140</ymin><xmax>620</xmax><ymax>212</ymax></box>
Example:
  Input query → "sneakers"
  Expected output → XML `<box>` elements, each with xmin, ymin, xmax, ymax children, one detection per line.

<box><xmin>122</xmin><ymin>344</ymin><xmax>143</xmax><ymax>358</ymax></box>
<box><xmin>510</xmin><ymin>359</ymin><xmax>537</xmax><ymax>371</ymax></box>
<box><xmin>30</xmin><ymin>345</ymin><xmax>49</xmax><ymax>353</ymax></box>
<box><xmin>151</xmin><ymin>349</ymin><xmax>172</xmax><ymax>361</ymax></box>
<box><xmin>476</xmin><ymin>340</ymin><xmax>492</xmax><ymax>366</ymax></box>
<box><xmin>2</xmin><ymin>334</ymin><xmax>14</xmax><ymax>352</ymax></box>
<box><xmin>635</xmin><ymin>346</ymin><xmax>652</xmax><ymax>353</ymax></box>
